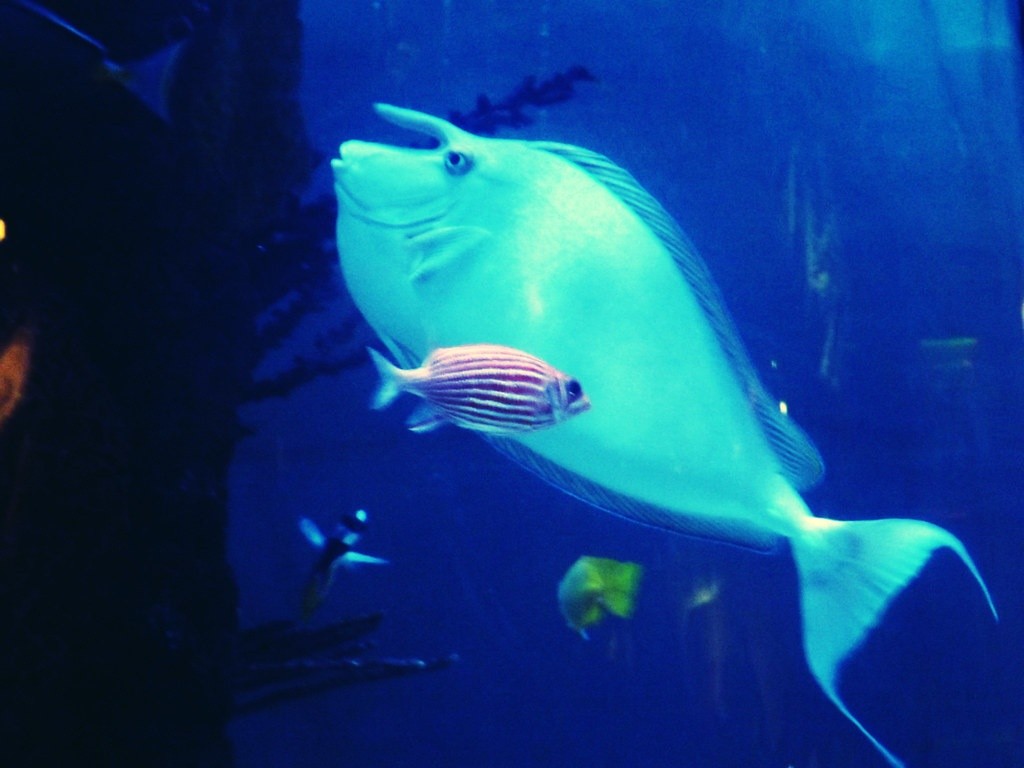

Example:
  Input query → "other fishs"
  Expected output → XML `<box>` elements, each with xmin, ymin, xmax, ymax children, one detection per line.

<box><xmin>219</xmin><ymin>97</ymin><xmax>1001</xmax><ymax>768</ymax></box>
